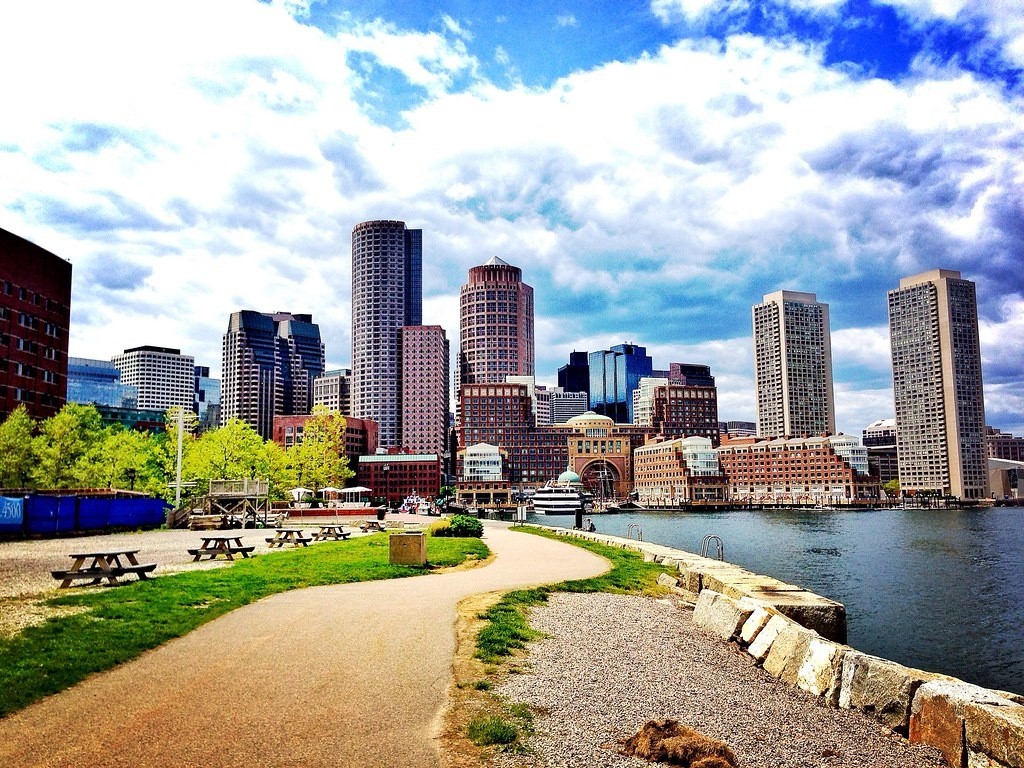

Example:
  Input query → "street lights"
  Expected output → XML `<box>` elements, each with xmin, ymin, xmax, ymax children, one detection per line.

<box><xmin>383</xmin><ymin>463</ymin><xmax>390</xmax><ymax>507</ymax></box>
<box><xmin>129</xmin><ymin>468</ymin><xmax>135</xmax><ymax>491</ymax></box>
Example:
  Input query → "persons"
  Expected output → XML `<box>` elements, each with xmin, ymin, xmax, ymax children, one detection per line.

<box><xmin>586</xmin><ymin>518</ymin><xmax>594</xmax><ymax>531</ymax></box>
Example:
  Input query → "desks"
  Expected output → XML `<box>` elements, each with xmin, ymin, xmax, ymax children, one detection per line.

<box><xmin>193</xmin><ymin>536</ymin><xmax>249</xmax><ymax>561</ymax></box>
<box><xmin>268</xmin><ymin>529</ymin><xmax>308</xmax><ymax>548</ymax></box>
<box><xmin>60</xmin><ymin>550</ymin><xmax>148</xmax><ymax>587</ymax></box>
<box><xmin>313</xmin><ymin>525</ymin><xmax>347</xmax><ymax>541</ymax></box>
<box><xmin>362</xmin><ymin>520</ymin><xmax>386</xmax><ymax>532</ymax></box>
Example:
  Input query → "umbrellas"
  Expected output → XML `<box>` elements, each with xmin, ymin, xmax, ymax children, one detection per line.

<box><xmin>318</xmin><ymin>487</ymin><xmax>340</xmax><ymax>500</ymax></box>
<box><xmin>289</xmin><ymin>487</ymin><xmax>313</xmax><ymax>502</ymax></box>
<box><xmin>336</xmin><ymin>486</ymin><xmax>372</xmax><ymax>503</ymax></box>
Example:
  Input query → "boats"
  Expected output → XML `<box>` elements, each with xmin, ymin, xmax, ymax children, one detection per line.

<box><xmin>530</xmin><ymin>478</ymin><xmax>593</xmax><ymax>515</ymax></box>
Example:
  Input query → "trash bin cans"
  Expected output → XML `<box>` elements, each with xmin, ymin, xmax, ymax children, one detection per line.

<box><xmin>477</xmin><ymin>508</ymin><xmax>485</xmax><ymax>519</ymax></box>
<box><xmin>377</xmin><ymin>509</ymin><xmax>385</xmax><ymax>520</ymax></box>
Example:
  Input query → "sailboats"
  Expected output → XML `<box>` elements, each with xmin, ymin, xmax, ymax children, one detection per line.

<box><xmin>584</xmin><ymin>451</ymin><xmax>621</xmax><ymax>514</ymax></box>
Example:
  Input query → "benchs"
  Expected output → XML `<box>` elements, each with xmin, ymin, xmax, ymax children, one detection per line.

<box><xmin>335</xmin><ymin>532</ymin><xmax>351</xmax><ymax>537</ymax></box>
<box><xmin>264</xmin><ymin>538</ymin><xmax>285</xmax><ymax>542</ymax></box>
<box><xmin>311</xmin><ymin>532</ymin><xmax>326</xmax><ymax>537</ymax></box>
<box><xmin>187</xmin><ymin>547</ymin><xmax>256</xmax><ymax>555</ymax></box>
<box><xmin>51</xmin><ymin>563</ymin><xmax>157</xmax><ymax>578</ymax></box>
<box><xmin>359</xmin><ymin>525</ymin><xmax>384</xmax><ymax>530</ymax></box>
<box><xmin>287</xmin><ymin>538</ymin><xmax>312</xmax><ymax>543</ymax></box>
